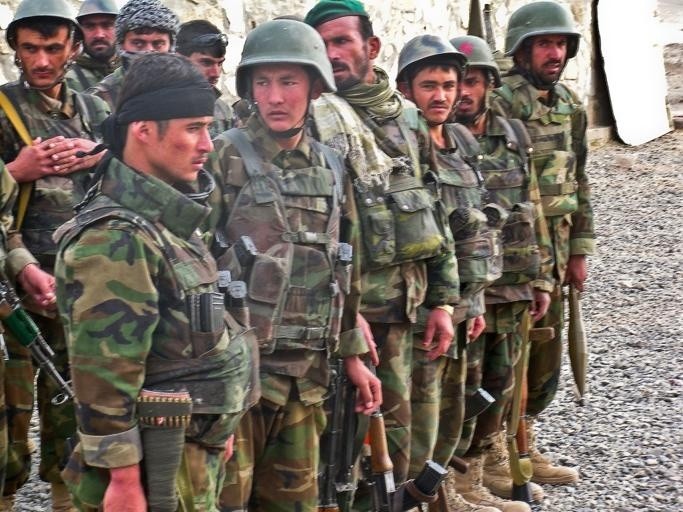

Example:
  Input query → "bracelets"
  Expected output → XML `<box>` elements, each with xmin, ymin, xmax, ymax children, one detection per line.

<box><xmin>434</xmin><ymin>302</ymin><xmax>454</xmax><ymax>317</ymax></box>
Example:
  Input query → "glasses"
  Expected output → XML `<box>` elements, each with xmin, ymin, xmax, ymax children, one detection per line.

<box><xmin>186</xmin><ymin>34</ymin><xmax>228</xmax><ymax>46</ymax></box>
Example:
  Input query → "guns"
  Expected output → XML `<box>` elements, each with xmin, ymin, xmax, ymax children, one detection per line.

<box><xmin>0</xmin><ymin>274</ymin><xmax>73</xmax><ymax>408</ymax></box>
<box><xmin>318</xmin><ymin>358</ymin><xmax>448</xmax><ymax>512</ymax></box>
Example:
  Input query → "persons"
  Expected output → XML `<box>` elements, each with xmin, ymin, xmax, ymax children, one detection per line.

<box><xmin>173</xmin><ymin>19</ymin><xmax>239</xmax><ymax>138</ymax></box>
<box><xmin>449</xmin><ymin>36</ymin><xmax>557</xmax><ymax>512</ymax></box>
<box><xmin>80</xmin><ymin>0</ymin><xmax>179</xmax><ymax>114</ymax></box>
<box><xmin>480</xmin><ymin>0</ymin><xmax>599</xmax><ymax>503</ymax></box>
<box><xmin>301</xmin><ymin>0</ymin><xmax>462</xmax><ymax>512</ymax></box>
<box><xmin>205</xmin><ymin>17</ymin><xmax>383</xmax><ymax>512</ymax></box>
<box><xmin>0</xmin><ymin>0</ymin><xmax>116</xmax><ymax>512</ymax></box>
<box><xmin>62</xmin><ymin>0</ymin><xmax>123</xmax><ymax>93</ymax></box>
<box><xmin>0</xmin><ymin>156</ymin><xmax>56</xmax><ymax>512</ymax></box>
<box><xmin>51</xmin><ymin>49</ymin><xmax>262</xmax><ymax>512</ymax></box>
<box><xmin>394</xmin><ymin>34</ymin><xmax>531</xmax><ymax>512</ymax></box>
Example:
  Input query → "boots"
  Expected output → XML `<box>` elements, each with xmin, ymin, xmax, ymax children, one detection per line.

<box><xmin>455</xmin><ymin>455</ymin><xmax>531</xmax><ymax>512</ymax></box>
<box><xmin>428</xmin><ymin>466</ymin><xmax>502</xmax><ymax>512</ymax></box>
<box><xmin>508</xmin><ymin>414</ymin><xmax>579</xmax><ymax>483</ymax></box>
<box><xmin>482</xmin><ymin>441</ymin><xmax>544</xmax><ymax>503</ymax></box>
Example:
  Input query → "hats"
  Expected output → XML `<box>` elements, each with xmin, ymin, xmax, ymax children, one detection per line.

<box><xmin>304</xmin><ymin>0</ymin><xmax>368</xmax><ymax>27</ymax></box>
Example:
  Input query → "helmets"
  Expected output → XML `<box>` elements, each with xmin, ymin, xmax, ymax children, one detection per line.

<box><xmin>236</xmin><ymin>19</ymin><xmax>337</xmax><ymax>100</ymax></box>
<box><xmin>396</xmin><ymin>35</ymin><xmax>468</xmax><ymax>81</ymax></box>
<box><xmin>76</xmin><ymin>1</ymin><xmax>121</xmax><ymax>18</ymax></box>
<box><xmin>450</xmin><ymin>36</ymin><xmax>501</xmax><ymax>88</ymax></box>
<box><xmin>6</xmin><ymin>0</ymin><xmax>86</xmax><ymax>50</ymax></box>
<box><xmin>504</xmin><ymin>1</ymin><xmax>581</xmax><ymax>57</ymax></box>
